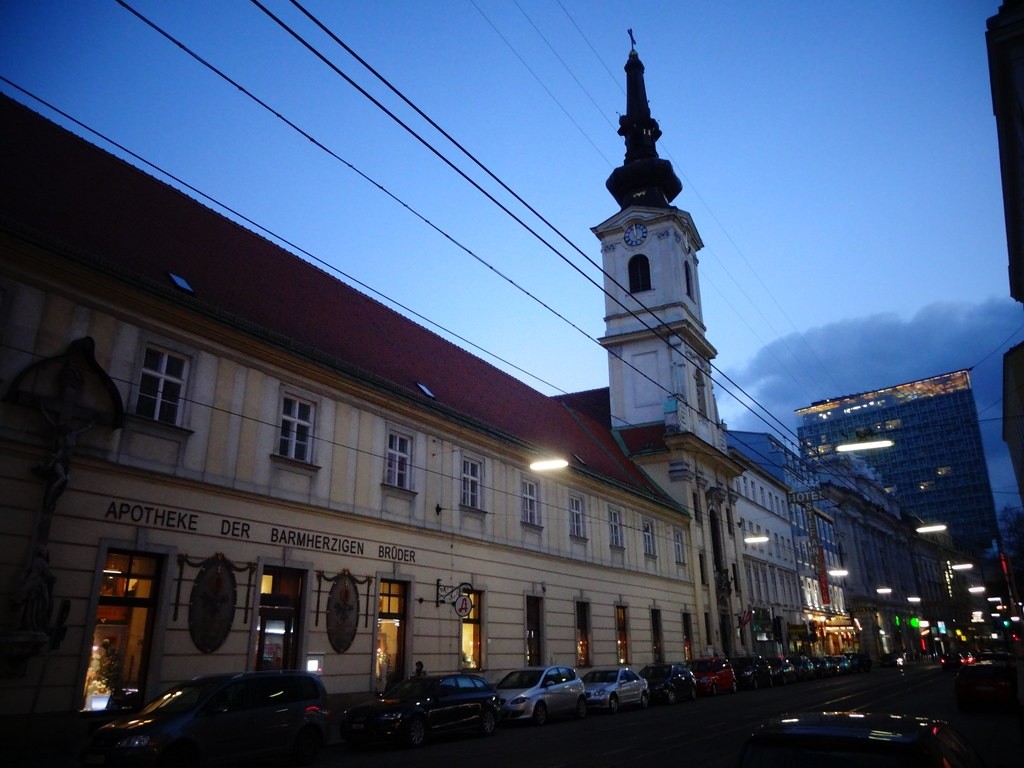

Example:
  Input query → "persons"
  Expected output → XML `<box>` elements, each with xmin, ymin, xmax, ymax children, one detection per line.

<box><xmin>416</xmin><ymin>661</ymin><xmax>427</xmax><ymax>676</ymax></box>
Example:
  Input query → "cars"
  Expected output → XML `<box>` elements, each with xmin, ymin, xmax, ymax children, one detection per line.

<box><xmin>491</xmin><ymin>666</ymin><xmax>587</xmax><ymax>724</ymax></box>
<box><xmin>735</xmin><ymin>709</ymin><xmax>980</xmax><ymax>767</ymax></box>
<box><xmin>954</xmin><ymin>649</ymin><xmax>1018</xmax><ymax>713</ymax></box>
<box><xmin>724</xmin><ymin>653</ymin><xmax>853</xmax><ymax>690</ymax></box>
<box><xmin>640</xmin><ymin>662</ymin><xmax>696</xmax><ymax>705</ymax></box>
<box><xmin>339</xmin><ymin>670</ymin><xmax>499</xmax><ymax>744</ymax></box>
<box><xmin>688</xmin><ymin>654</ymin><xmax>734</xmax><ymax>697</ymax></box>
<box><xmin>576</xmin><ymin>668</ymin><xmax>649</xmax><ymax>711</ymax></box>
<box><xmin>81</xmin><ymin>670</ymin><xmax>329</xmax><ymax>768</ymax></box>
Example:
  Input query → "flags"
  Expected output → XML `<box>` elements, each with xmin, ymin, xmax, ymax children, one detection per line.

<box><xmin>736</xmin><ymin>608</ymin><xmax>752</xmax><ymax>629</ymax></box>
